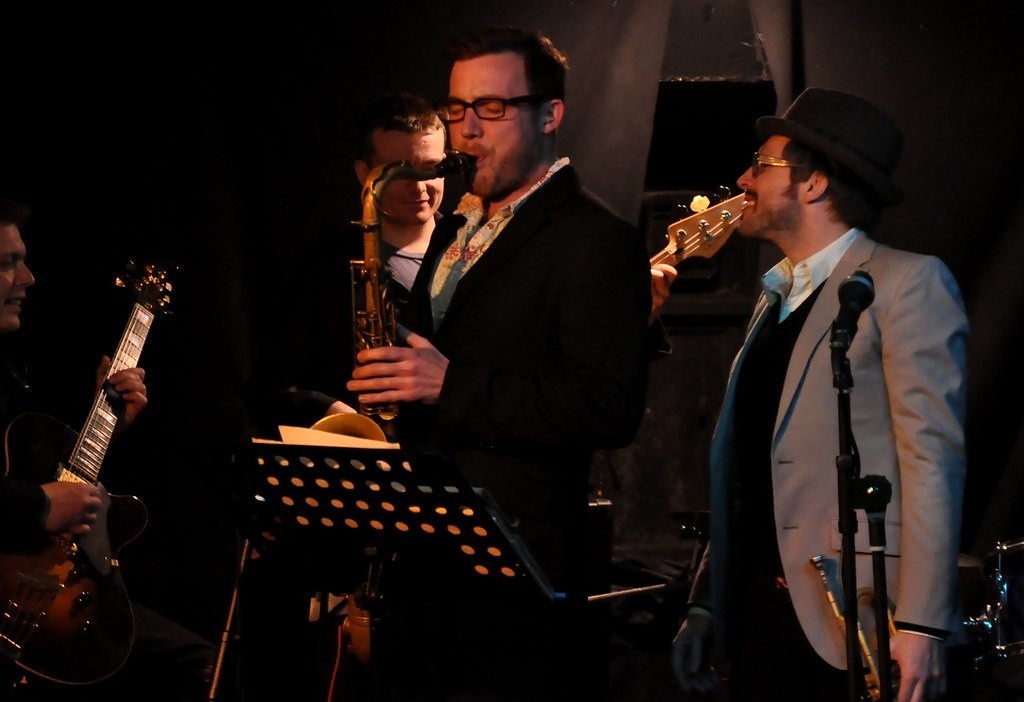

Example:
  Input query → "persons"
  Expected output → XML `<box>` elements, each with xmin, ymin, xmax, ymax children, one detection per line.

<box><xmin>0</xmin><ymin>206</ymin><xmax>215</xmax><ymax>702</ymax></box>
<box><xmin>671</xmin><ymin>89</ymin><xmax>969</xmax><ymax>702</ymax></box>
<box><xmin>302</xmin><ymin>94</ymin><xmax>677</xmax><ymax>397</ymax></box>
<box><xmin>345</xmin><ymin>25</ymin><xmax>651</xmax><ymax>702</ymax></box>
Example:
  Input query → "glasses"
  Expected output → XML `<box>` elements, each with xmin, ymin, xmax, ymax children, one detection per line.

<box><xmin>436</xmin><ymin>95</ymin><xmax>534</xmax><ymax>122</ymax></box>
<box><xmin>752</xmin><ymin>152</ymin><xmax>808</xmax><ymax>176</ymax></box>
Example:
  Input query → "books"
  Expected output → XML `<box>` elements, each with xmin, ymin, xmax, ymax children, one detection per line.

<box><xmin>276</xmin><ymin>414</ymin><xmax>401</xmax><ymax>449</ymax></box>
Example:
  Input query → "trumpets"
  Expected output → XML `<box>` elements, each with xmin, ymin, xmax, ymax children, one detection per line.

<box><xmin>808</xmin><ymin>556</ymin><xmax>899</xmax><ymax>702</ymax></box>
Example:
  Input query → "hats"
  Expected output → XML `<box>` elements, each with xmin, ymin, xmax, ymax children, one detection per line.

<box><xmin>755</xmin><ymin>87</ymin><xmax>906</xmax><ymax>207</ymax></box>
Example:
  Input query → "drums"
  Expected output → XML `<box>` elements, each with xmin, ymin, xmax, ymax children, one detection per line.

<box><xmin>981</xmin><ymin>640</ymin><xmax>1024</xmax><ymax>702</ymax></box>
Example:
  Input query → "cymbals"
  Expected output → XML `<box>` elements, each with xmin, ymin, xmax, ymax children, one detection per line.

<box><xmin>671</xmin><ymin>509</ymin><xmax>711</xmax><ymax>532</ymax></box>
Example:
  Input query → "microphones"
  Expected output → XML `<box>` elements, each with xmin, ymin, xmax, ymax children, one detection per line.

<box><xmin>831</xmin><ymin>270</ymin><xmax>876</xmax><ymax>380</ymax></box>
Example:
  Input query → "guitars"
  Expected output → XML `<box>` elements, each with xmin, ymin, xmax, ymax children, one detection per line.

<box><xmin>0</xmin><ymin>259</ymin><xmax>179</xmax><ymax>702</ymax></box>
<box><xmin>647</xmin><ymin>185</ymin><xmax>755</xmax><ymax>289</ymax></box>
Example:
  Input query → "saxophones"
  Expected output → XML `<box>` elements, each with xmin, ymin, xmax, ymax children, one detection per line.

<box><xmin>311</xmin><ymin>148</ymin><xmax>476</xmax><ymax>669</ymax></box>
